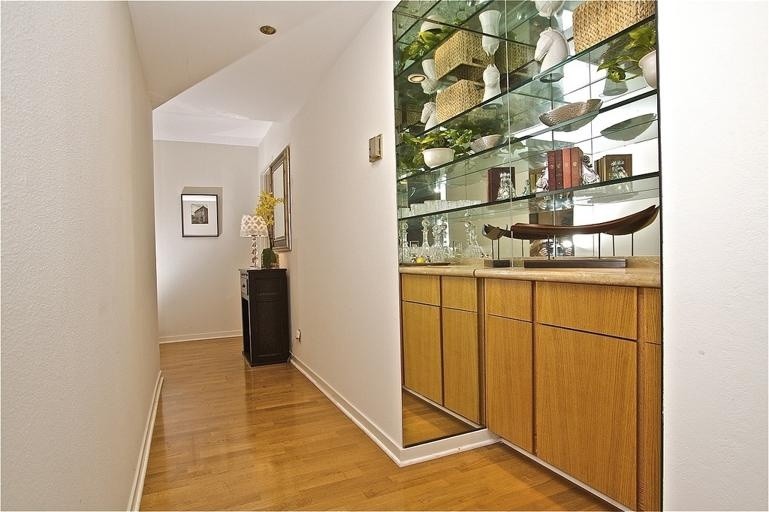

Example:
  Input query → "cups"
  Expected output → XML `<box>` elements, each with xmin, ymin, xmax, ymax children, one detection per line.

<box><xmin>452</xmin><ymin>240</ymin><xmax>463</xmax><ymax>263</ymax></box>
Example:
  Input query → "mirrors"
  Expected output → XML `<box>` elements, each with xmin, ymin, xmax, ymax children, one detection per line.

<box><xmin>485</xmin><ymin>0</ymin><xmax>660</xmax><ymax>259</ymax></box>
<box><xmin>269</xmin><ymin>146</ymin><xmax>291</xmax><ymax>251</ymax></box>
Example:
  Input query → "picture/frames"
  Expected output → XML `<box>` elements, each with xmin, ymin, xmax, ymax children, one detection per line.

<box><xmin>181</xmin><ymin>194</ymin><xmax>219</xmax><ymax>237</ymax></box>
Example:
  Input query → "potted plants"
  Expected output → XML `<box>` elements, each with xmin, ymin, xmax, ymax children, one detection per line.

<box><xmin>256</xmin><ymin>191</ymin><xmax>282</xmax><ymax>267</ymax></box>
<box><xmin>399</xmin><ymin>127</ymin><xmax>477</xmax><ymax>175</ymax></box>
<box><xmin>409</xmin><ymin>15</ymin><xmax>463</xmax><ymax>61</ymax></box>
<box><xmin>596</xmin><ymin>21</ymin><xmax>659</xmax><ymax>90</ymax></box>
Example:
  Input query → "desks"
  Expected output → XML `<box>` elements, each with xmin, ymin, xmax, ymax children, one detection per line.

<box><xmin>238</xmin><ymin>268</ymin><xmax>289</xmax><ymax>368</ymax></box>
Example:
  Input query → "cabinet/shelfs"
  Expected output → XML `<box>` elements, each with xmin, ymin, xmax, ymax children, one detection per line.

<box><xmin>473</xmin><ymin>0</ymin><xmax>663</xmax><ymax>512</ymax></box>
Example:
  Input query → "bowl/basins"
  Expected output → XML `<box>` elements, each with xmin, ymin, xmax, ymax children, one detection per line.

<box><xmin>537</xmin><ymin>98</ymin><xmax>606</xmax><ymax>134</ymax></box>
<box><xmin>600</xmin><ymin>112</ymin><xmax>656</xmax><ymax>141</ymax></box>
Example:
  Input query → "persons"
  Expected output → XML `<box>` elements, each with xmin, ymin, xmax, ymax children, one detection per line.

<box><xmin>529</xmin><ymin>239</ymin><xmax>573</xmax><ymax>256</ymax></box>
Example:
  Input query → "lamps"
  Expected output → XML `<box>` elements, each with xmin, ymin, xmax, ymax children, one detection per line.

<box><xmin>240</xmin><ymin>215</ymin><xmax>269</xmax><ymax>270</ymax></box>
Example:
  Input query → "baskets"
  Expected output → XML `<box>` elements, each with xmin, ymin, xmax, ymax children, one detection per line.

<box><xmin>573</xmin><ymin>0</ymin><xmax>657</xmax><ymax>55</ymax></box>
<box><xmin>435</xmin><ymin>31</ymin><xmax>495</xmax><ymax>80</ymax></box>
<box><xmin>435</xmin><ymin>80</ymin><xmax>484</xmax><ymax>121</ymax></box>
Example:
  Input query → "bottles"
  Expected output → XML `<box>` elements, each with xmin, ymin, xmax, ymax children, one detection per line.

<box><xmin>462</xmin><ymin>221</ymin><xmax>484</xmax><ymax>261</ymax></box>
<box><xmin>401</xmin><ymin>220</ymin><xmax>437</xmax><ymax>264</ymax></box>
<box><xmin>432</xmin><ymin>216</ymin><xmax>451</xmax><ymax>263</ymax></box>
